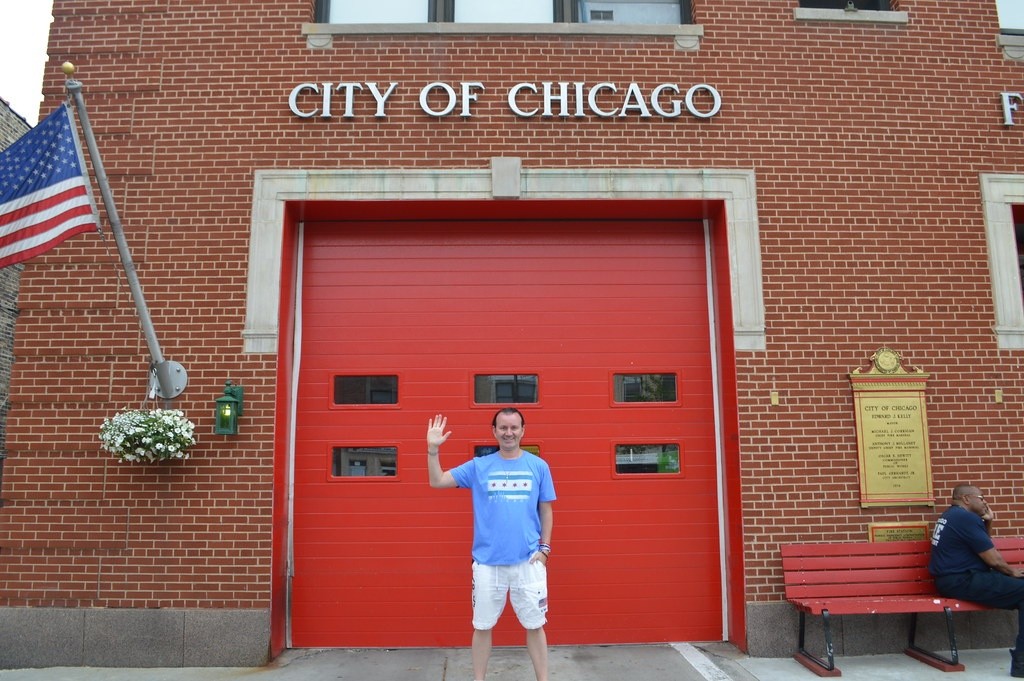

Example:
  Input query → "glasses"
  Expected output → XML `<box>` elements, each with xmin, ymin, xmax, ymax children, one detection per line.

<box><xmin>977</xmin><ymin>495</ymin><xmax>984</xmax><ymax>500</ymax></box>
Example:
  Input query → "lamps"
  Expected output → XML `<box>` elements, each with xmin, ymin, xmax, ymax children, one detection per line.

<box><xmin>214</xmin><ymin>380</ymin><xmax>244</xmax><ymax>435</ymax></box>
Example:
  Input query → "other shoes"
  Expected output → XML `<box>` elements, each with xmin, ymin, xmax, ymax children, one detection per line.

<box><xmin>1009</xmin><ymin>649</ymin><xmax>1024</xmax><ymax>677</ymax></box>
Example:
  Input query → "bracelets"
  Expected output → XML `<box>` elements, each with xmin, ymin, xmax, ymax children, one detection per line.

<box><xmin>537</xmin><ymin>542</ymin><xmax>552</xmax><ymax>558</ymax></box>
<box><xmin>426</xmin><ymin>450</ymin><xmax>438</xmax><ymax>458</ymax></box>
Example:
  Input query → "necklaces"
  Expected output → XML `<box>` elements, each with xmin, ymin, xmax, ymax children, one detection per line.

<box><xmin>497</xmin><ymin>449</ymin><xmax>520</xmax><ymax>483</ymax></box>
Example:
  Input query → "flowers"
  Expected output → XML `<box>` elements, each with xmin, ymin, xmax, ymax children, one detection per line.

<box><xmin>98</xmin><ymin>405</ymin><xmax>197</xmax><ymax>463</ymax></box>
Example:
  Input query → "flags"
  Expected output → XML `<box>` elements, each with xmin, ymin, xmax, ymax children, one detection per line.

<box><xmin>0</xmin><ymin>80</ymin><xmax>180</xmax><ymax>394</ymax></box>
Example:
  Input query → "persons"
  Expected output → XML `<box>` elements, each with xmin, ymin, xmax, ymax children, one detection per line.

<box><xmin>426</xmin><ymin>406</ymin><xmax>557</xmax><ymax>681</ymax></box>
<box><xmin>930</xmin><ymin>486</ymin><xmax>1023</xmax><ymax>675</ymax></box>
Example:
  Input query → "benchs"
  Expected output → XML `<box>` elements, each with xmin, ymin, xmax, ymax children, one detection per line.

<box><xmin>780</xmin><ymin>538</ymin><xmax>1024</xmax><ymax>677</ymax></box>
